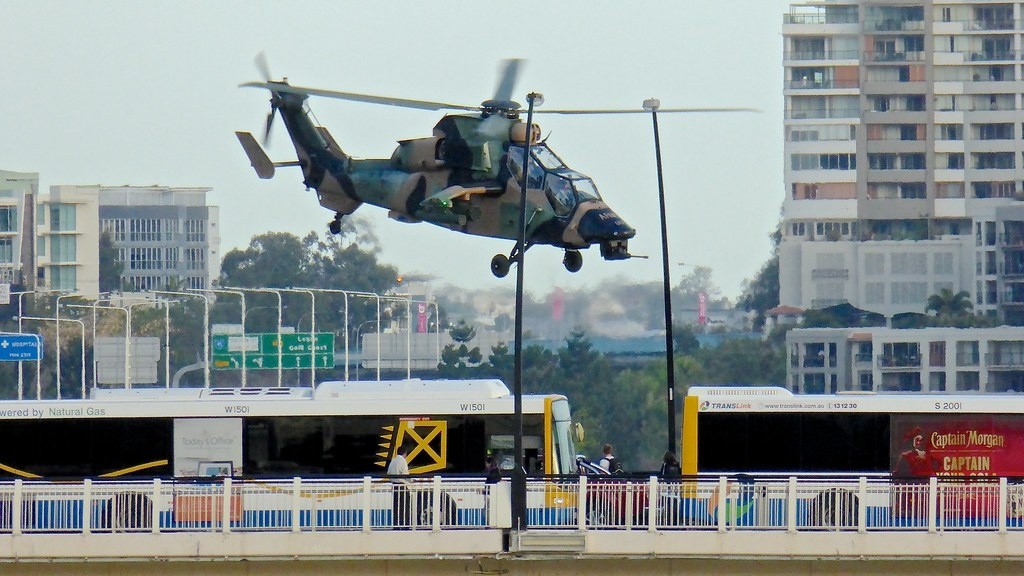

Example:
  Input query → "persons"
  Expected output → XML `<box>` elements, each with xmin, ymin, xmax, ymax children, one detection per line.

<box><xmin>659</xmin><ymin>451</ymin><xmax>681</xmax><ymax>478</ymax></box>
<box><xmin>387</xmin><ymin>446</ymin><xmax>414</xmax><ymax>531</ymax></box>
<box><xmin>482</xmin><ymin>457</ymin><xmax>501</xmax><ymax>529</ymax></box>
<box><xmin>893</xmin><ymin>435</ymin><xmax>937</xmax><ymax>477</ymax></box>
<box><xmin>511</xmin><ymin>150</ymin><xmax>536</xmax><ymax>181</ymax></box>
<box><xmin>600</xmin><ymin>444</ymin><xmax>624</xmax><ymax>475</ymax></box>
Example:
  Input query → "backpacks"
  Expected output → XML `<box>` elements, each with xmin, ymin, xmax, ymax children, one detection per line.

<box><xmin>664</xmin><ymin>463</ymin><xmax>682</xmax><ymax>485</ymax></box>
<box><xmin>602</xmin><ymin>456</ymin><xmax>622</xmax><ymax>476</ymax></box>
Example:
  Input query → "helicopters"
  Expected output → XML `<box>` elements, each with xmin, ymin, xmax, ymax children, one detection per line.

<box><xmin>234</xmin><ymin>58</ymin><xmax>764</xmax><ymax>279</ymax></box>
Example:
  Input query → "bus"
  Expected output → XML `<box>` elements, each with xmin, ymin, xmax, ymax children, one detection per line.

<box><xmin>0</xmin><ymin>381</ymin><xmax>583</xmax><ymax>551</ymax></box>
<box><xmin>679</xmin><ymin>385</ymin><xmax>1024</xmax><ymax>530</ymax></box>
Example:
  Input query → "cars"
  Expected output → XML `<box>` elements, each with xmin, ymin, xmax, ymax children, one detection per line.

<box><xmin>573</xmin><ymin>455</ymin><xmax>648</xmax><ymax>530</ymax></box>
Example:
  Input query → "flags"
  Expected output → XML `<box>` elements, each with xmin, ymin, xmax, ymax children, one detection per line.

<box><xmin>418</xmin><ymin>303</ymin><xmax>427</xmax><ymax>333</ymax></box>
<box><xmin>698</xmin><ymin>291</ymin><xmax>708</xmax><ymax>324</ymax></box>
<box><xmin>551</xmin><ymin>293</ymin><xmax>564</xmax><ymax>320</ymax></box>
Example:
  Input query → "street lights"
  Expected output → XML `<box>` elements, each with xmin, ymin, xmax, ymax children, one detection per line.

<box><xmin>68</xmin><ymin>302</ymin><xmax>127</xmax><ymax>388</ymax></box>
<box><xmin>11</xmin><ymin>314</ymin><xmax>86</xmax><ymax>398</ymax></box>
<box><xmin>0</xmin><ymin>290</ymin><xmax>38</xmax><ymax>295</ymax></box>
<box><xmin>143</xmin><ymin>290</ymin><xmax>210</xmax><ymax>387</ymax></box>
<box><xmin>111</xmin><ymin>295</ymin><xmax>170</xmax><ymax>388</ymax></box>
<box><xmin>324</xmin><ymin>291</ymin><xmax>380</xmax><ymax>381</ymax></box>
<box><xmin>54</xmin><ymin>290</ymin><xmax>110</xmax><ymax>398</ymax></box>
<box><xmin>350</xmin><ymin>294</ymin><xmax>422</xmax><ymax>380</ymax></box>
<box><xmin>282</xmin><ymin>286</ymin><xmax>350</xmax><ymax>381</ymax></box>
<box><xmin>179</xmin><ymin>288</ymin><xmax>246</xmax><ymax>388</ymax></box>
<box><xmin>16</xmin><ymin>287</ymin><xmax>79</xmax><ymax>396</ymax></box>
<box><xmin>93</xmin><ymin>296</ymin><xmax>148</xmax><ymax>388</ymax></box>
<box><xmin>251</xmin><ymin>288</ymin><xmax>316</xmax><ymax>391</ymax></box>
<box><xmin>386</xmin><ymin>299</ymin><xmax>440</xmax><ymax>369</ymax></box>
<box><xmin>218</xmin><ymin>285</ymin><xmax>282</xmax><ymax>388</ymax></box>
